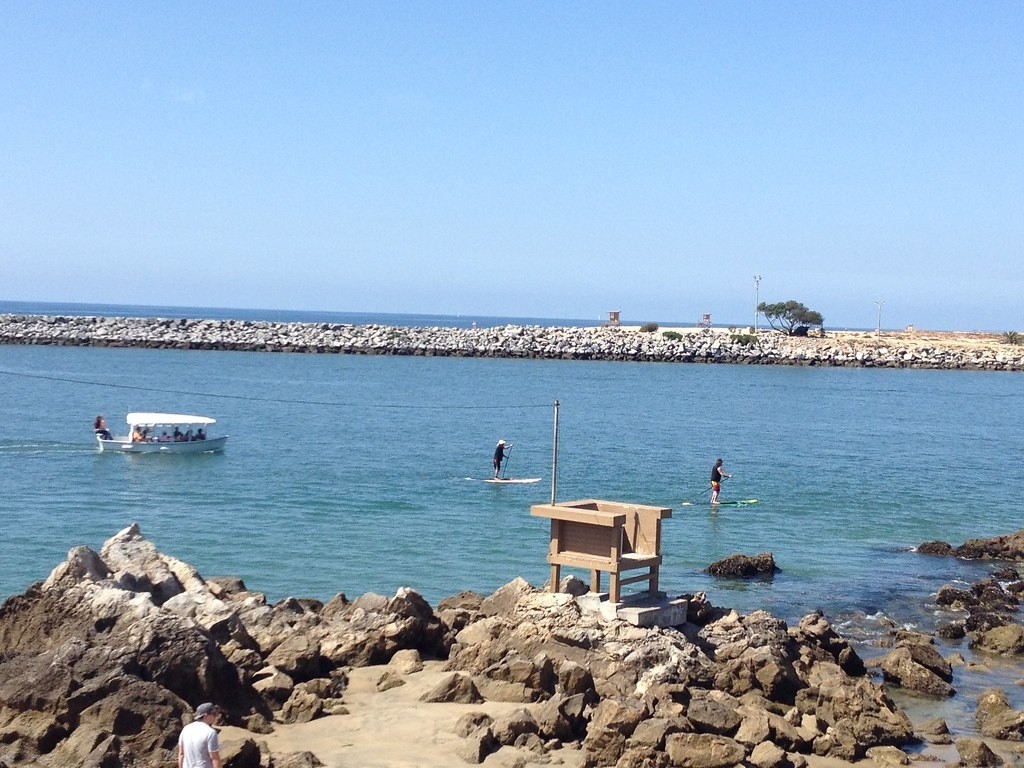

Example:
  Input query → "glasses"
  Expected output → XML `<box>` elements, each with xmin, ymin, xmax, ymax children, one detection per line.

<box><xmin>208</xmin><ymin>712</ymin><xmax>217</xmax><ymax>717</ymax></box>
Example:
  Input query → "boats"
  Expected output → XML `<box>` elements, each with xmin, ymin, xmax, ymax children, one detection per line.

<box><xmin>96</xmin><ymin>413</ymin><xmax>228</xmax><ymax>455</ymax></box>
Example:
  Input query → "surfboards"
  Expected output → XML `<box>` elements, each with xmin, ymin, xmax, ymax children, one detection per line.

<box><xmin>682</xmin><ymin>499</ymin><xmax>757</xmax><ymax>508</ymax></box>
<box><xmin>465</xmin><ymin>477</ymin><xmax>542</xmax><ymax>483</ymax></box>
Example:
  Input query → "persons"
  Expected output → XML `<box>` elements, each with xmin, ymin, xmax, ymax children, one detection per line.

<box><xmin>174</xmin><ymin>427</ymin><xmax>183</xmax><ymax>439</ymax></box>
<box><xmin>133</xmin><ymin>427</ymin><xmax>147</xmax><ymax>442</ymax></box>
<box><xmin>195</xmin><ymin>428</ymin><xmax>205</xmax><ymax>441</ymax></box>
<box><xmin>493</xmin><ymin>440</ymin><xmax>512</xmax><ymax>480</ymax></box>
<box><xmin>94</xmin><ymin>416</ymin><xmax>113</xmax><ymax>440</ymax></box>
<box><xmin>178</xmin><ymin>703</ymin><xmax>223</xmax><ymax>768</ymax></box>
<box><xmin>160</xmin><ymin>432</ymin><xmax>169</xmax><ymax>441</ymax></box>
<box><xmin>143</xmin><ymin>428</ymin><xmax>149</xmax><ymax>437</ymax></box>
<box><xmin>186</xmin><ymin>430</ymin><xmax>194</xmax><ymax>441</ymax></box>
<box><xmin>710</xmin><ymin>459</ymin><xmax>732</xmax><ymax>505</ymax></box>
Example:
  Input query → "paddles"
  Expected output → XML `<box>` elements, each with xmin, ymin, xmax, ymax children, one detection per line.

<box><xmin>696</xmin><ymin>472</ymin><xmax>735</xmax><ymax>496</ymax></box>
<box><xmin>502</xmin><ymin>444</ymin><xmax>513</xmax><ymax>479</ymax></box>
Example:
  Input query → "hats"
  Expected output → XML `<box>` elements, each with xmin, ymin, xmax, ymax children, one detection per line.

<box><xmin>195</xmin><ymin>703</ymin><xmax>213</xmax><ymax>719</ymax></box>
<box><xmin>497</xmin><ymin>440</ymin><xmax>505</xmax><ymax>446</ymax></box>
